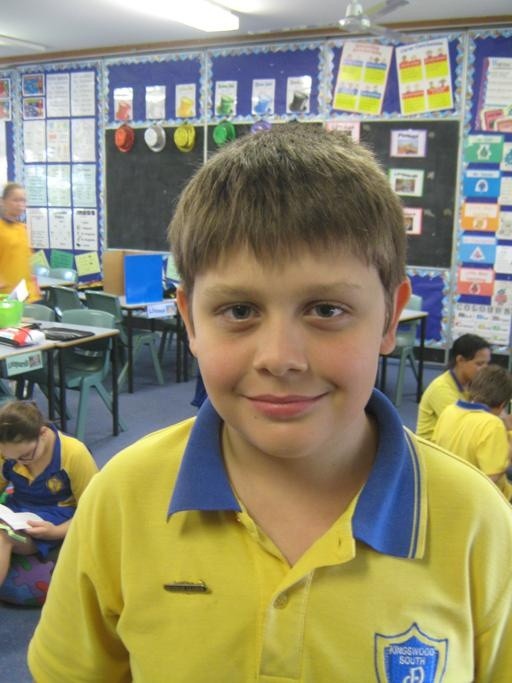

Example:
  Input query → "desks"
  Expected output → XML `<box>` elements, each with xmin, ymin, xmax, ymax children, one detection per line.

<box><xmin>380</xmin><ymin>308</ymin><xmax>429</xmax><ymax>403</ymax></box>
<box><xmin>118</xmin><ymin>294</ymin><xmax>188</xmax><ymax>394</ymax></box>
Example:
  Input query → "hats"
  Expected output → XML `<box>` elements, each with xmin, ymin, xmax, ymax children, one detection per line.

<box><xmin>144</xmin><ymin>125</ymin><xmax>166</xmax><ymax>153</ymax></box>
<box><xmin>216</xmin><ymin>96</ymin><xmax>234</xmax><ymax>114</ymax></box>
<box><xmin>178</xmin><ymin>97</ymin><xmax>193</xmax><ymax>117</ymax></box>
<box><xmin>212</xmin><ymin>122</ymin><xmax>236</xmax><ymax>147</ymax></box>
<box><xmin>115</xmin><ymin>125</ymin><xmax>135</xmax><ymax>152</ymax></box>
<box><xmin>149</xmin><ymin>96</ymin><xmax>164</xmax><ymax>118</ymax></box>
<box><xmin>116</xmin><ymin>102</ymin><xmax>130</xmax><ymax>120</ymax></box>
<box><xmin>173</xmin><ymin>125</ymin><xmax>195</xmax><ymax>152</ymax></box>
<box><xmin>251</xmin><ymin>121</ymin><xmax>272</xmax><ymax>134</ymax></box>
<box><xmin>253</xmin><ymin>95</ymin><xmax>272</xmax><ymax>116</ymax></box>
<box><xmin>289</xmin><ymin>92</ymin><xmax>308</xmax><ymax>112</ymax></box>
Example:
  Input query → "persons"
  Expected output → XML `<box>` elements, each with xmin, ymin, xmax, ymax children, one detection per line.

<box><xmin>431</xmin><ymin>365</ymin><xmax>512</xmax><ymax>506</ymax></box>
<box><xmin>26</xmin><ymin>121</ymin><xmax>512</xmax><ymax>683</ymax></box>
<box><xmin>0</xmin><ymin>399</ymin><xmax>100</xmax><ymax>586</ymax></box>
<box><xmin>416</xmin><ymin>334</ymin><xmax>512</xmax><ymax>442</ymax></box>
<box><xmin>0</xmin><ymin>182</ymin><xmax>44</xmax><ymax>304</ymax></box>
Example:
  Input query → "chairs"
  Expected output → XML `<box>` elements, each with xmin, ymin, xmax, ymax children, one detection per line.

<box><xmin>0</xmin><ymin>264</ymin><xmax>164</xmax><ymax>444</ymax></box>
<box><xmin>391</xmin><ymin>294</ymin><xmax>424</xmax><ymax>408</ymax></box>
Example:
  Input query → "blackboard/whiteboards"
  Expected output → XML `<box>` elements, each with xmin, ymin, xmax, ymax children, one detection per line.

<box><xmin>104</xmin><ymin>118</ymin><xmax>460</xmax><ymax>270</ymax></box>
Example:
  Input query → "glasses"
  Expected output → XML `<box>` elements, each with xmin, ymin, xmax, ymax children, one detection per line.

<box><xmin>1</xmin><ymin>435</ymin><xmax>38</xmax><ymax>463</ymax></box>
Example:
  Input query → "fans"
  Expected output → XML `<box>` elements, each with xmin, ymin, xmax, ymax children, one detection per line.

<box><xmin>333</xmin><ymin>1</ymin><xmax>417</xmax><ymax>44</ymax></box>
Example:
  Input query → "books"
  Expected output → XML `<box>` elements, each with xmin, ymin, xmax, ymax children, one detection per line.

<box><xmin>0</xmin><ymin>504</ymin><xmax>44</xmax><ymax>531</ymax></box>
